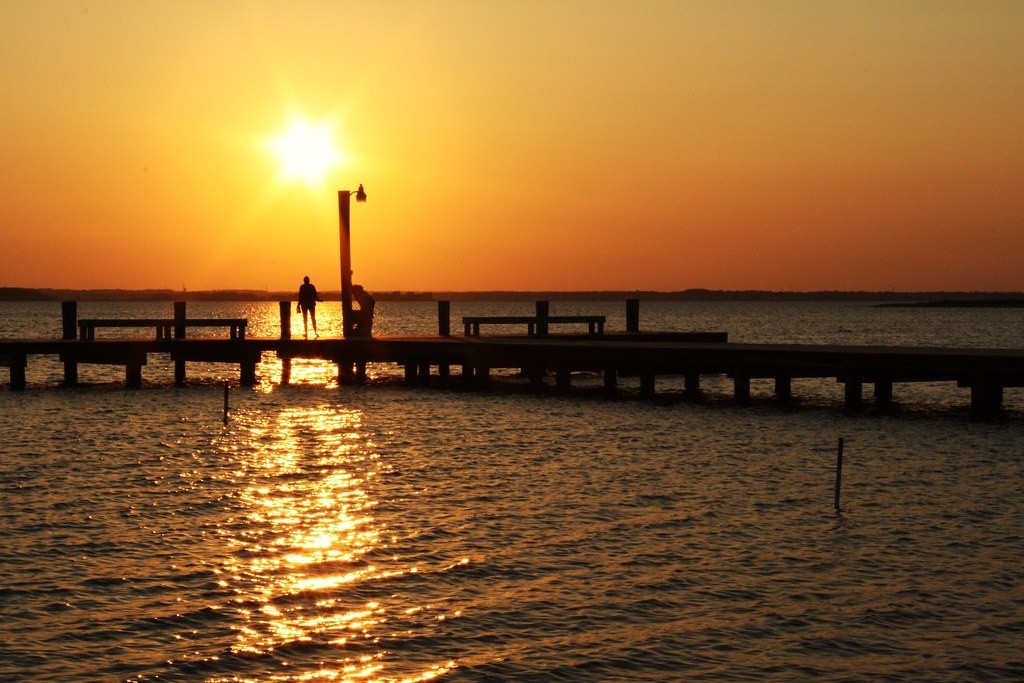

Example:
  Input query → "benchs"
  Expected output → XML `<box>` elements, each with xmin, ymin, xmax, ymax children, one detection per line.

<box><xmin>461</xmin><ymin>317</ymin><xmax>608</xmax><ymax>334</ymax></box>
<box><xmin>76</xmin><ymin>318</ymin><xmax>248</xmax><ymax>340</ymax></box>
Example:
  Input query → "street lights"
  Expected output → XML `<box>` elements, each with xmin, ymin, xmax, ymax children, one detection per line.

<box><xmin>337</xmin><ymin>184</ymin><xmax>367</xmax><ymax>337</ymax></box>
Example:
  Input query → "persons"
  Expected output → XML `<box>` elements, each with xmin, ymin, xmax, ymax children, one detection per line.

<box><xmin>297</xmin><ymin>276</ymin><xmax>323</xmax><ymax>337</ymax></box>
<box><xmin>352</xmin><ymin>284</ymin><xmax>375</xmax><ymax>336</ymax></box>
